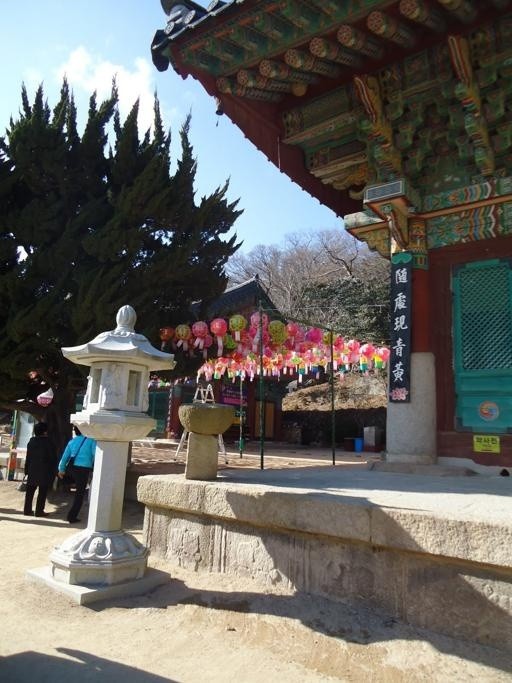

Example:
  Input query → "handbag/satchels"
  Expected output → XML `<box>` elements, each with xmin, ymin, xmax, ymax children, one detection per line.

<box><xmin>17</xmin><ymin>475</ymin><xmax>28</xmax><ymax>491</ymax></box>
<box><xmin>65</xmin><ymin>456</ymin><xmax>74</xmax><ymax>471</ymax></box>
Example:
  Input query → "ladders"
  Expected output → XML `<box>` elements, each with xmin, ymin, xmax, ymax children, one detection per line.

<box><xmin>172</xmin><ymin>382</ymin><xmax>229</xmax><ymax>464</ymax></box>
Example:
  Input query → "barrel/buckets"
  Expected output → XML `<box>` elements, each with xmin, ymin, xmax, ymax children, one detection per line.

<box><xmin>343</xmin><ymin>436</ymin><xmax>352</xmax><ymax>453</ymax></box>
<box><xmin>353</xmin><ymin>436</ymin><xmax>363</xmax><ymax>453</ymax></box>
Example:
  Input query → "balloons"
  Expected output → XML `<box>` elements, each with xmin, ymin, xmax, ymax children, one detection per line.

<box><xmin>160</xmin><ymin>312</ymin><xmax>391</xmax><ymax>382</ymax></box>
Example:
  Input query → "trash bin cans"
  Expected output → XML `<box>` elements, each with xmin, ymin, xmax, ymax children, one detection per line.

<box><xmin>353</xmin><ymin>438</ymin><xmax>364</xmax><ymax>452</ymax></box>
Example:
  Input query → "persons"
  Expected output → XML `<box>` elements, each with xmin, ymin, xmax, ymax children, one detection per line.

<box><xmin>57</xmin><ymin>425</ymin><xmax>97</xmax><ymax>523</ymax></box>
<box><xmin>23</xmin><ymin>423</ymin><xmax>57</xmax><ymax>517</ymax></box>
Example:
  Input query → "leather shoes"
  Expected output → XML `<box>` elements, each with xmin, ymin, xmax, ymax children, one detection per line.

<box><xmin>23</xmin><ymin>510</ymin><xmax>81</xmax><ymax>524</ymax></box>
